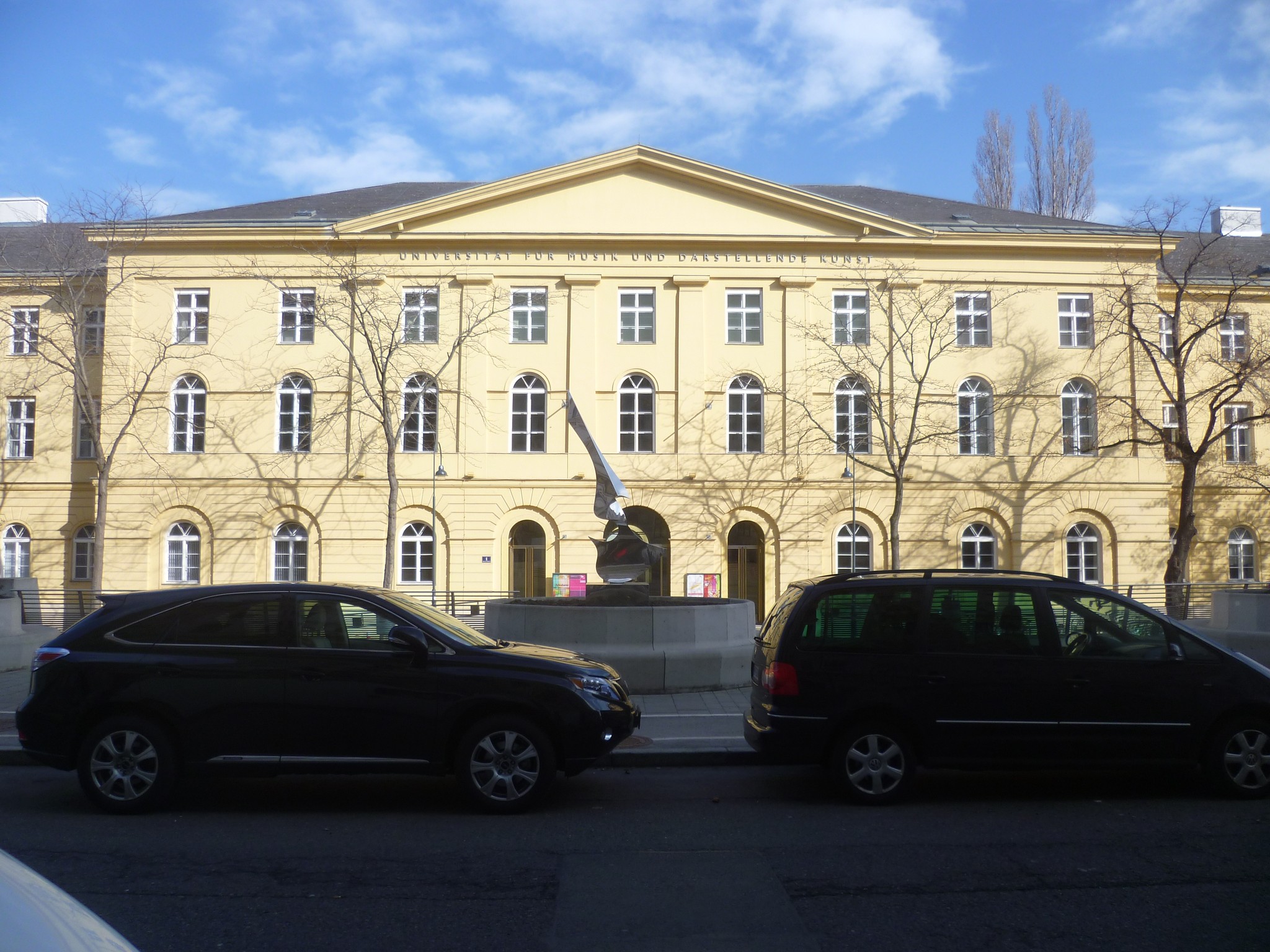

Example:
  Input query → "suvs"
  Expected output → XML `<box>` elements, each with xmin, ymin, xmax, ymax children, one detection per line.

<box><xmin>746</xmin><ymin>569</ymin><xmax>1270</xmax><ymax>807</ymax></box>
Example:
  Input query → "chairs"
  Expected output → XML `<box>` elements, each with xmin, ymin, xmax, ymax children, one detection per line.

<box><xmin>292</xmin><ymin>603</ymin><xmax>336</xmax><ymax>649</ymax></box>
<box><xmin>937</xmin><ymin>597</ymin><xmax>970</xmax><ymax>640</ymax></box>
<box><xmin>973</xmin><ymin>601</ymin><xmax>997</xmax><ymax>644</ymax></box>
<box><xmin>994</xmin><ymin>598</ymin><xmax>1031</xmax><ymax>646</ymax></box>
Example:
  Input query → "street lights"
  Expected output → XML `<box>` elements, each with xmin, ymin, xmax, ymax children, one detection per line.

<box><xmin>840</xmin><ymin>443</ymin><xmax>855</xmax><ymax>576</ymax></box>
<box><xmin>430</xmin><ymin>443</ymin><xmax>449</xmax><ymax>606</ymax></box>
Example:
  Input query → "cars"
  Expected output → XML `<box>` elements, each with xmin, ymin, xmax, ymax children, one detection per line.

<box><xmin>13</xmin><ymin>584</ymin><xmax>642</xmax><ymax>817</ymax></box>
